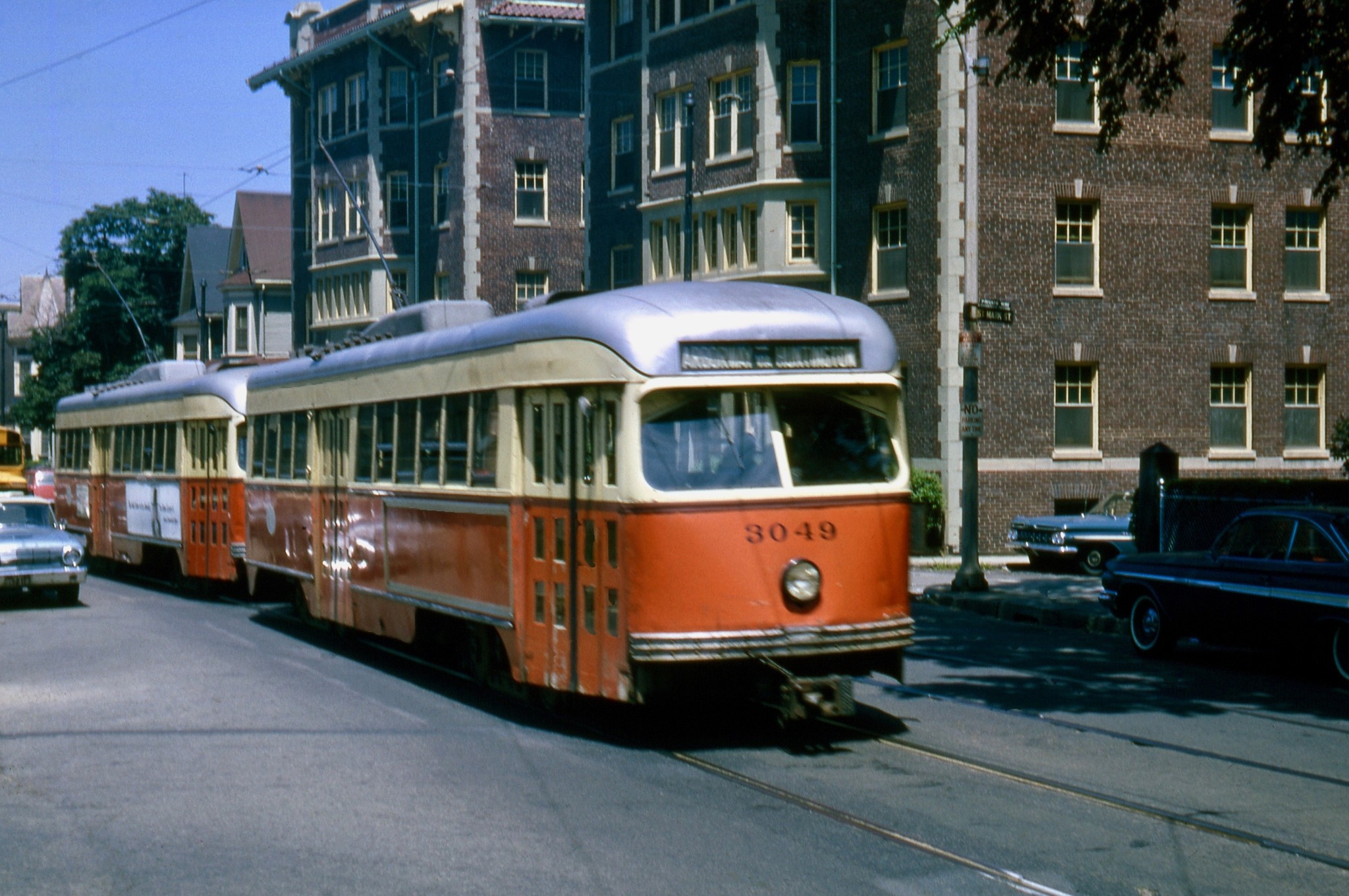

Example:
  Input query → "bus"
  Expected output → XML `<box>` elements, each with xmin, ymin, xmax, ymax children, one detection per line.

<box><xmin>49</xmin><ymin>356</ymin><xmax>267</xmax><ymax>592</ymax></box>
<box><xmin>242</xmin><ymin>279</ymin><xmax>920</xmax><ymax>737</ymax></box>
<box><xmin>1</xmin><ymin>427</ymin><xmax>34</xmax><ymax>496</ymax></box>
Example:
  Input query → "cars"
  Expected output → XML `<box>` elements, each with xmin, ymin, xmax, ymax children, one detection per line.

<box><xmin>0</xmin><ymin>491</ymin><xmax>89</xmax><ymax>606</ymax></box>
<box><xmin>1004</xmin><ymin>490</ymin><xmax>1139</xmax><ymax>577</ymax></box>
<box><xmin>1095</xmin><ymin>507</ymin><xmax>1349</xmax><ymax>707</ymax></box>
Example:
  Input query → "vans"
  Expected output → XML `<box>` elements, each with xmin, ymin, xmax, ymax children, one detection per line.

<box><xmin>25</xmin><ymin>468</ymin><xmax>56</xmax><ymax>505</ymax></box>
